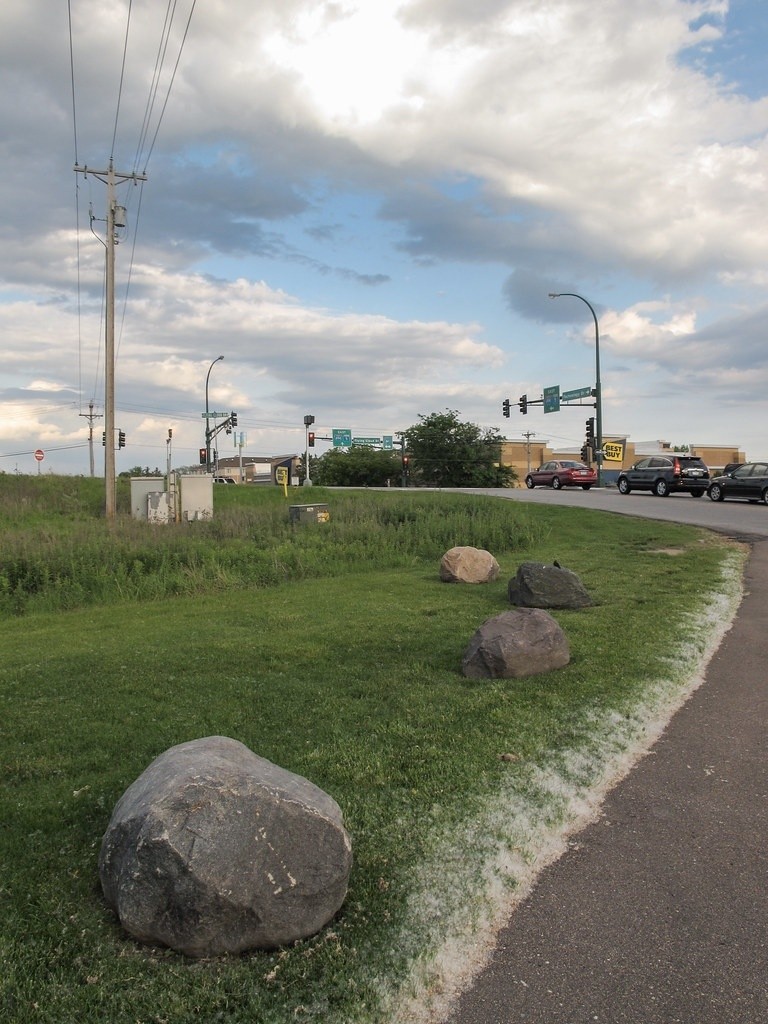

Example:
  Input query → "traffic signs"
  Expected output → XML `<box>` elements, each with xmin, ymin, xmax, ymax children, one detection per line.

<box><xmin>544</xmin><ymin>385</ymin><xmax>561</xmax><ymax>413</ymax></box>
<box><xmin>383</xmin><ymin>436</ymin><xmax>392</xmax><ymax>450</ymax></box>
<box><xmin>562</xmin><ymin>386</ymin><xmax>590</xmax><ymax>401</ymax></box>
<box><xmin>332</xmin><ymin>429</ymin><xmax>352</xmax><ymax>447</ymax></box>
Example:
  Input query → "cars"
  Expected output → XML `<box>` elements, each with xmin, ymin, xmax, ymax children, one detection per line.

<box><xmin>707</xmin><ymin>461</ymin><xmax>768</xmax><ymax>504</ymax></box>
<box><xmin>524</xmin><ymin>460</ymin><xmax>597</xmax><ymax>490</ymax></box>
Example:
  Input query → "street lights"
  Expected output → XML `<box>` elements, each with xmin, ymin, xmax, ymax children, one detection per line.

<box><xmin>304</xmin><ymin>415</ymin><xmax>315</xmax><ymax>486</ymax></box>
<box><xmin>549</xmin><ymin>293</ymin><xmax>602</xmax><ymax>487</ymax></box>
<box><xmin>205</xmin><ymin>355</ymin><xmax>224</xmax><ymax>474</ymax></box>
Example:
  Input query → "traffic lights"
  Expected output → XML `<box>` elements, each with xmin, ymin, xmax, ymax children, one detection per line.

<box><xmin>403</xmin><ymin>457</ymin><xmax>408</xmax><ymax>469</ymax></box>
<box><xmin>200</xmin><ymin>449</ymin><xmax>206</xmax><ymax>464</ymax></box>
<box><xmin>103</xmin><ymin>432</ymin><xmax>106</xmax><ymax>446</ymax></box>
<box><xmin>585</xmin><ymin>417</ymin><xmax>595</xmax><ymax>449</ymax></box>
<box><xmin>226</xmin><ymin>422</ymin><xmax>232</xmax><ymax>434</ymax></box>
<box><xmin>580</xmin><ymin>445</ymin><xmax>586</xmax><ymax>462</ymax></box>
<box><xmin>503</xmin><ymin>399</ymin><xmax>509</xmax><ymax>418</ymax></box>
<box><xmin>119</xmin><ymin>431</ymin><xmax>126</xmax><ymax>447</ymax></box>
<box><xmin>231</xmin><ymin>411</ymin><xmax>237</xmax><ymax>427</ymax></box>
<box><xmin>401</xmin><ymin>470</ymin><xmax>409</xmax><ymax>477</ymax></box>
<box><xmin>519</xmin><ymin>395</ymin><xmax>527</xmax><ymax>414</ymax></box>
<box><xmin>213</xmin><ymin>451</ymin><xmax>218</xmax><ymax>459</ymax></box>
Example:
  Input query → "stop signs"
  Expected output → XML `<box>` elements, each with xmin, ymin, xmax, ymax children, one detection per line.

<box><xmin>34</xmin><ymin>449</ymin><xmax>44</xmax><ymax>461</ymax></box>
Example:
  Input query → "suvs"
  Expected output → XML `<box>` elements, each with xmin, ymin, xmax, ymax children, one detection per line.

<box><xmin>723</xmin><ymin>463</ymin><xmax>743</xmax><ymax>475</ymax></box>
<box><xmin>616</xmin><ymin>454</ymin><xmax>710</xmax><ymax>498</ymax></box>
<box><xmin>212</xmin><ymin>478</ymin><xmax>237</xmax><ymax>484</ymax></box>
